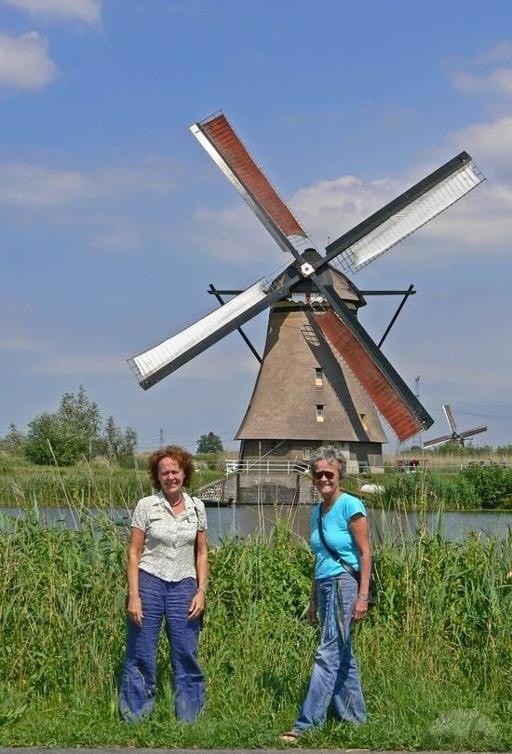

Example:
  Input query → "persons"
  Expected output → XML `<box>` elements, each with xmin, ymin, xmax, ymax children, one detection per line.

<box><xmin>117</xmin><ymin>443</ymin><xmax>210</xmax><ymax>722</ymax></box>
<box><xmin>276</xmin><ymin>444</ymin><xmax>373</xmax><ymax>742</ymax></box>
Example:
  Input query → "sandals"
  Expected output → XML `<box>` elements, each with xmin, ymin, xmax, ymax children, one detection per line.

<box><xmin>281</xmin><ymin>732</ymin><xmax>298</xmax><ymax>741</ymax></box>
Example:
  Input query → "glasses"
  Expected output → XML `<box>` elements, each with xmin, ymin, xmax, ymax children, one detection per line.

<box><xmin>313</xmin><ymin>471</ymin><xmax>334</xmax><ymax>480</ymax></box>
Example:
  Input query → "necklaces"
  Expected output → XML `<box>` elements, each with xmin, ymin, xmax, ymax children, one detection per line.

<box><xmin>164</xmin><ymin>493</ymin><xmax>183</xmax><ymax>507</ymax></box>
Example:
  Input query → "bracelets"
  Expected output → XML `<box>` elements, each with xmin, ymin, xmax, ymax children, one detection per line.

<box><xmin>196</xmin><ymin>588</ymin><xmax>207</xmax><ymax>595</ymax></box>
<box><xmin>357</xmin><ymin>594</ymin><xmax>368</xmax><ymax>600</ymax></box>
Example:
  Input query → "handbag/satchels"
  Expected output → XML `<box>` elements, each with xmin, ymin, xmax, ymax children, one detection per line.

<box><xmin>357</xmin><ymin>571</ymin><xmax>376</xmax><ymax>610</ymax></box>
